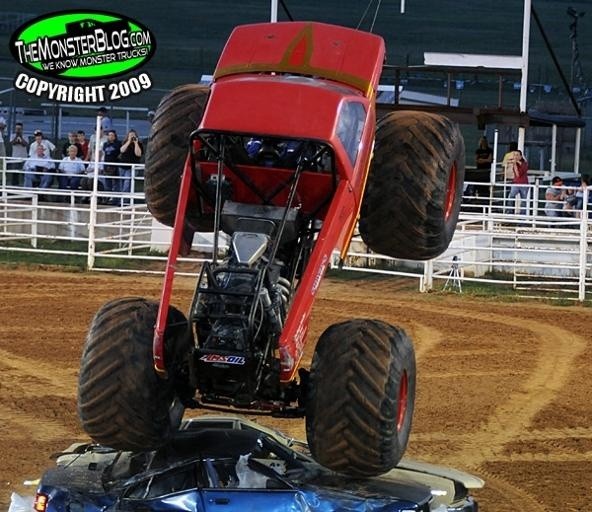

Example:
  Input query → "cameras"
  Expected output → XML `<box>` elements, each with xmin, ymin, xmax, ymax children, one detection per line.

<box><xmin>132</xmin><ymin>137</ymin><xmax>136</xmax><ymax>142</ymax></box>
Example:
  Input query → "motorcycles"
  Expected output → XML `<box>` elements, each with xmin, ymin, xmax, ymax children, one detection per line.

<box><xmin>79</xmin><ymin>23</ymin><xmax>465</xmax><ymax>476</ymax></box>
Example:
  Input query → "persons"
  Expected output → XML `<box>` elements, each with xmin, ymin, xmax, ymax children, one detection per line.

<box><xmin>475</xmin><ymin>133</ymin><xmax>493</xmax><ymax>167</ymax></box>
<box><xmin>574</xmin><ymin>173</ymin><xmax>589</xmax><ymax>218</ymax></box>
<box><xmin>503</xmin><ymin>150</ymin><xmax>529</xmax><ymax>227</ymax></box>
<box><xmin>543</xmin><ymin>176</ymin><xmax>563</xmax><ymax>226</ymax></box>
<box><xmin>1</xmin><ymin>106</ymin><xmax>148</xmax><ymax>205</ymax></box>
<box><xmin>500</xmin><ymin>140</ymin><xmax>519</xmax><ymax>212</ymax></box>
<box><xmin>560</xmin><ymin>187</ymin><xmax>574</xmax><ymax>228</ymax></box>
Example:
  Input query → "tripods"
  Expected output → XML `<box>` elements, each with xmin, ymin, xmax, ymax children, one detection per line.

<box><xmin>441</xmin><ymin>263</ymin><xmax>462</xmax><ymax>294</ymax></box>
